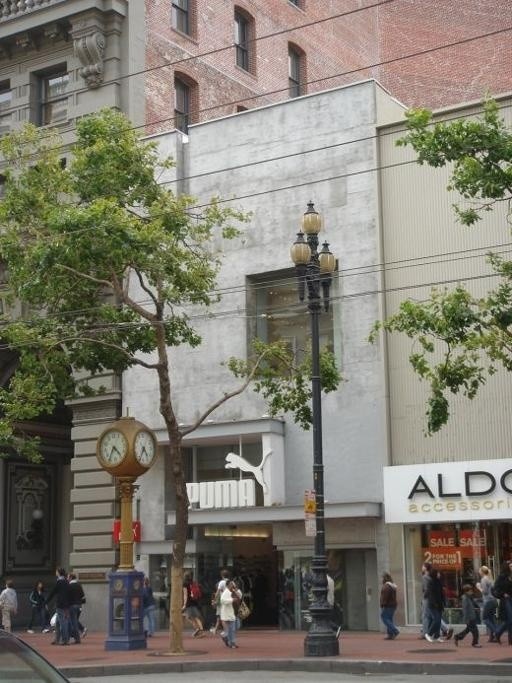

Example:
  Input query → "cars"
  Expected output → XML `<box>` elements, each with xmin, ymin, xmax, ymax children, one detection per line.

<box><xmin>0</xmin><ymin>629</ymin><xmax>90</xmax><ymax>683</ymax></box>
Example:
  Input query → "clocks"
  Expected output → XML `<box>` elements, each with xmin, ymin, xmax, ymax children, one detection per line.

<box><xmin>97</xmin><ymin>426</ymin><xmax>131</xmax><ymax>468</ymax></box>
<box><xmin>132</xmin><ymin>426</ymin><xmax>158</xmax><ymax>468</ymax></box>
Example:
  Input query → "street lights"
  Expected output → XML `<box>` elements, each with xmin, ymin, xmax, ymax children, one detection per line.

<box><xmin>291</xmin><ymin>200</ymin><xmax>346</xmax><ymax>658</ymax></box>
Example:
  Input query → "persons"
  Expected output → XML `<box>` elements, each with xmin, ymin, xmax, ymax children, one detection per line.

<box><xmin>153</xmin><ymin>574</ymin><xmax>170</xmax><ymax>619</ymax></box>
<box><xmin>1</xmin><ymin>578</ymin><xmax>18</xmax><ymax>635</ymax></box>
<box><xmin>379</xmin><ymin>571</ymin><xmax>400</xmax><ymax>640</ymax></box>
<box><xmin>183</xmin><ymin>568</ymin><xmax>272</xmax><ymax>649</ymax></box>
<box><xmin>26</xmin><ymin>579</ymin><xmax>53</xmax><ymax>635</ymax></box>
<box><xmin>416</xmin><ymin>560</ymin><xmax>512</xmax><ymax>648</ymax></box>
<box><xmin>326</xmin><ymin>574</ymin><xmax>341</xmax><ymax>640</ymax></box>
<box><xmin>131</xmin><ymin>576</ymin><xmax>157</xmax><ymax>637</ymax></box>
<box><xmin>41</xmin><ymin>566</ymin><xmax>88</xmax><ymax>647</ymax></box>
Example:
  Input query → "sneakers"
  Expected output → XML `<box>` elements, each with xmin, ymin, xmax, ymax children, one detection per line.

<box><xmin>27</xmin><ymin>629</ymin><xmax>49</xmax><ymax>633</ymax></box>
<box><xmin>51</xmin><ymin>627</ymin><xmax>88</xmax><ymax>645</ymax></box>
<box><xmin>486</xmin><ymin>633</ymin><xmax>512</xmax><ymax>645</ymax></box>
<box><xmin>210</xmin><ymin>628</ymin><xmax>239</xmax><ymax>648</ymax></box>
<box><xmin>193</xmin><ymin>629</ymin><xmax>204</xmax><ymax>638</ymax></box>
<box><xmin>384</xmin><ymin>632</ymin><xmax>400</xmax><ymax>640</ymax></box>
<box><xmin>424</xmin><ymin>629</ymin><xmax>458</xmax><ymax>647</ymax></box>
<box><xmin>472</xmin><ymin>643</ymin><xmax>481</xmax><ymax>648</ymax></box>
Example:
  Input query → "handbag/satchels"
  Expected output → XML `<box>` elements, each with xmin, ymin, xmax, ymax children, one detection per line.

<box><xmin>191</xmin><ymin>586</ymin><xmax>200</xmax><ymax>601</ymax></box>
<box><xmin>237</xmin><ymin>601</ymin><xmax>250</xmax><ymax>619</ymax></box>
